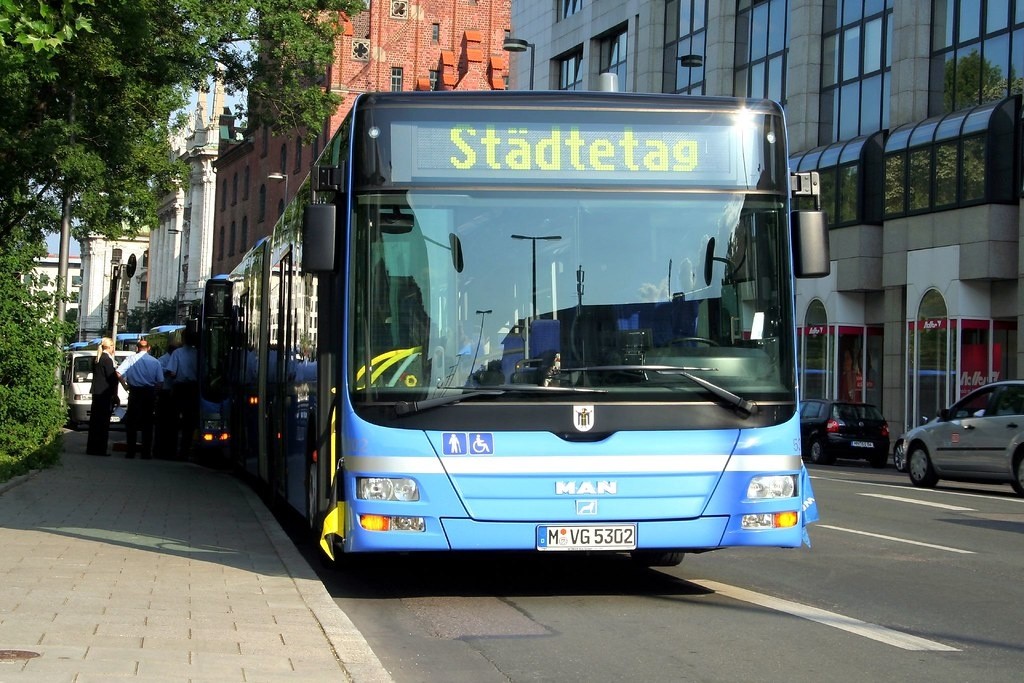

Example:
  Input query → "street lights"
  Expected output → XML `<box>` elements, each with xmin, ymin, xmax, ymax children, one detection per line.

<box><xmin>267</xmin><ymin>171</ymin><xmax>288</xmax><ymax>210</ymax></box>
<box><xmin>168</xmin><ymin>228</ymin><xmax>182</xmax><ymax>324</ymax></box>
<box><xmin>503</xmin><ymin>38</ymin><xmax>536</xmax><ymax>90</ymax></box>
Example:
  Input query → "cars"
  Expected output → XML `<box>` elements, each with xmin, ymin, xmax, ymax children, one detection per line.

<box><xmin>893</xmin><ymin>379</ymin><xmax>1024</xmax><ymax>497</ymax></box>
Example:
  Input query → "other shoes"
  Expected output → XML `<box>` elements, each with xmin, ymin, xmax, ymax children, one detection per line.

<box><xmin>140</xmin><ymin>456</ymin><xmax>153</xmax><ymax>460</ymax></box>
<box><xmin>124</xmin><ymin>454</ymin><xmax>135</xmax><ymax>460</ymax></box>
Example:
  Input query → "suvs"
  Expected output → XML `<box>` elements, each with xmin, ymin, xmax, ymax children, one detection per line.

<box><xmin>799</xmin><ymin>398</ymin><xmax>890</xmax><ymax>466</ymax></box>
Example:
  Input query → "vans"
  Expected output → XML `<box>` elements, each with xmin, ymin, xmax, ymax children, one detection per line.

<box><xmin>61</xmin><ymin>349</ymin><xmax>138</xmax><ymax>430</ymax></box>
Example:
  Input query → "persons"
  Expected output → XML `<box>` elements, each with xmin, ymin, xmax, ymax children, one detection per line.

<box><xmin>84</xmin><ymin>328</ymin><xmax>198</xmax><ymax>459</ymax></box>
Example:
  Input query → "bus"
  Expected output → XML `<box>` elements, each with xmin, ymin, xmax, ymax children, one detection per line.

<box><xmin>62</xmin><ymin>325</ymin><xmax>186</xmax><ymax>354</ymax></box>
<box><xmin>186</xmin><ymin>86</ymin><xmax>832</xmax><ymax>582</ymax></box>
